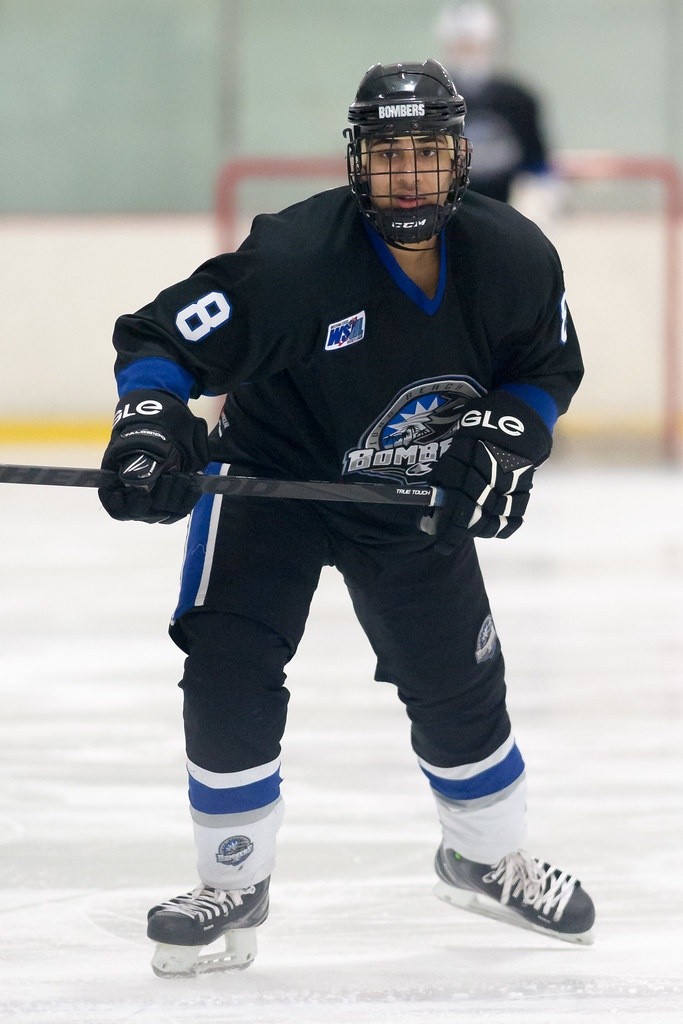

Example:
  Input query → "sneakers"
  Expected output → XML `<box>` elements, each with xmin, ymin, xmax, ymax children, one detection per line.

<box><xmin>146</xmin><ymin>875</ymin><xmax>271</xmax><ymax>980</ymax></box>
<box><xmin>432</xmin><ymin>841</ymin><xmax>602</xmax><ymax>947</ymax></box>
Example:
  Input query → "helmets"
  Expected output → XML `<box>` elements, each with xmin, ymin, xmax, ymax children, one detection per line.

<box><xmin>348</xmin><ymin>59</ymin><xmax>467</xmax><ymax>138</ymax></box>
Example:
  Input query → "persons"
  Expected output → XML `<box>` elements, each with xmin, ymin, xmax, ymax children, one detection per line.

<box><xmin>428</xmin><ymin>1</ymin><xmax>553</xmax><ymax>222</ymax></box>
<box><xmin>97</xmin><ymin>57</ymin><xmax>597</xmax><ymax>978</ymax></box>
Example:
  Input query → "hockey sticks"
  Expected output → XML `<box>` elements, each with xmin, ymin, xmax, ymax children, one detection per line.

<box><xmin>0</xmin><ymin>465</ymin><xmax>446</xmax><ymax>506</ymax></box>
